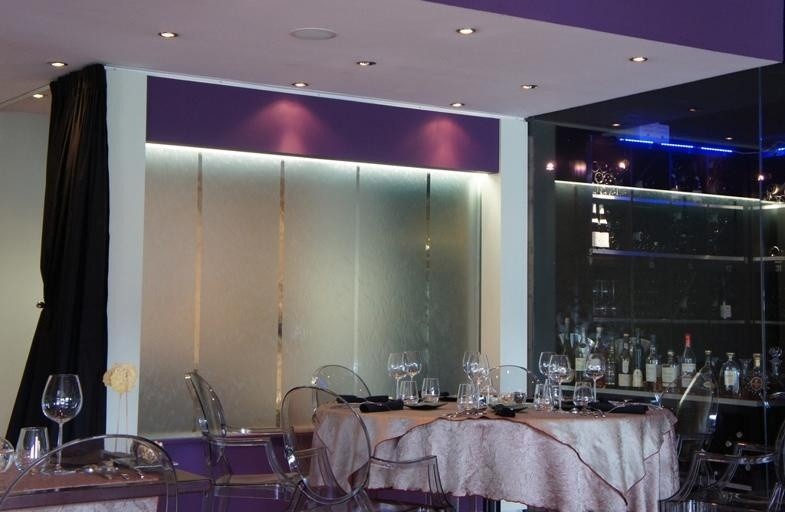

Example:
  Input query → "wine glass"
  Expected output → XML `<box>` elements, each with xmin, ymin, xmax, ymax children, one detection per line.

<box><xmin>41</xmin><ymin>373</ymin><xmax>83</xmax><ymax>475</ymax></box>
<box><xmin>385</xmin><ymin>351</ymin><xmax>608</xmax><ymax>418</ymax></box>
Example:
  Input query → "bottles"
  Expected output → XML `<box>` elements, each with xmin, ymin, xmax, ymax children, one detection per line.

<box><xmin>574</xmin><ymin>324</ymin><xmax>782</xmax><ymax>402</ymax></box>
<box><xmin>591</xmin><ymin>203</ymin><xmax>617</xmax><ymax>251</ymax></box>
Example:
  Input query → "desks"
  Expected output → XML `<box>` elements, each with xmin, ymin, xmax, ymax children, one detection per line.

<box><xmin>308</xmin><ymin>400</ymin><xmax>681</xmax><ymax>512</ymax></box>
<box><xmin>0</xmin><ymin>449</ymin><xmax>212</xmax><ymax>512</ymax></box>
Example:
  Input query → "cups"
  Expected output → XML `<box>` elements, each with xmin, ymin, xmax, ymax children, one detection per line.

<box><xmin>14</xmin><ymin>426</ymin><xmax>49</xmax><ymax>475</ymax></box>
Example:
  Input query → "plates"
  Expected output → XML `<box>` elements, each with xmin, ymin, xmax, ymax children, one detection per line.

<box><xmin>114</xmin><ymin>457</ymin><xmax>178</xmax><ymax>472</ymax></box>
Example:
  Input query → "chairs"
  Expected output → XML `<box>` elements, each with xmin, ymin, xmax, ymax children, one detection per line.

<box><xmin>280</xmin><ymin>386</ymin><xmax>457</xmax><ymax>512</ymax></box>
<box><xmin>659</xmin><ymin>389</ymin><xmax>785</xmax><ymax>511</ymax></box>
<box><xmin>312</xmin><ymin>365</ymin><xmax>372</xmax><ymax>407</ymax></box>
<box><xmin>0</xmin><ymin>434</ymin><xmax>178</xmax><ymax>510</ymax></box>
<box><xmin>183</xmin><ymin>368</ymin><xmax>302</xmax><ymax>510</ymax></box>
<box><xmin>470</xmin><ymin>355</ymin><xmax>715</xmax><ymax>512</ymax></box>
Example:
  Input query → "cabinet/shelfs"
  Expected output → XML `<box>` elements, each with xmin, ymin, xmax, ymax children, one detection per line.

<box><xmin>586</xmin><ymin>192</ymin><xmax>785</xmax><ymax>329</ymax></box>
